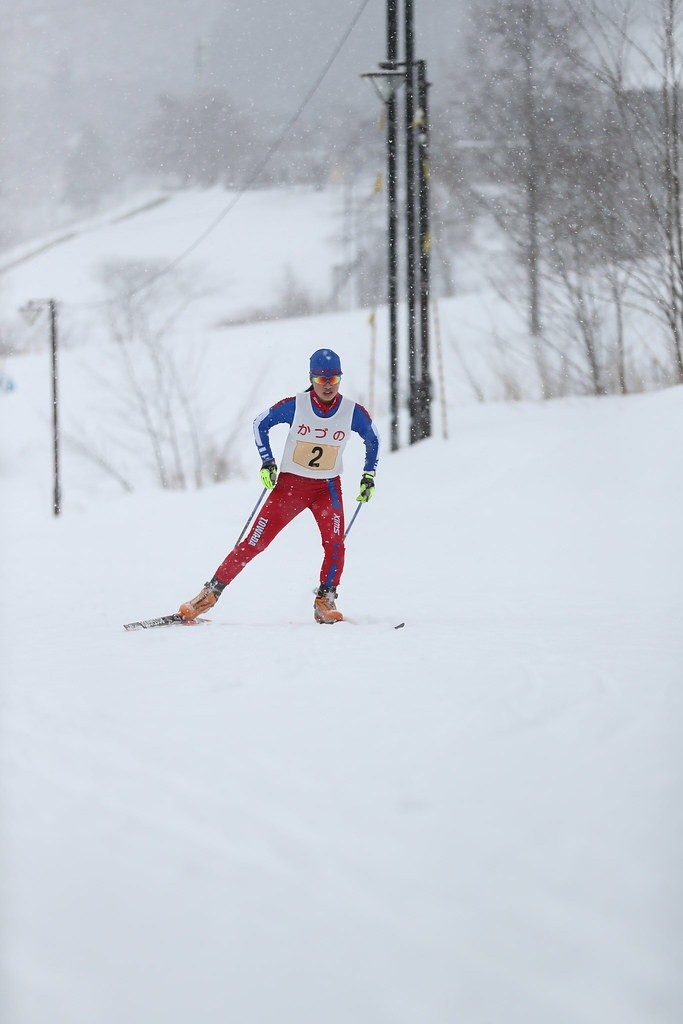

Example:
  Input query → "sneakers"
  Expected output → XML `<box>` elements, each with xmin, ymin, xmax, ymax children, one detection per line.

<box><xmin>313</xmin><ymin>585</ymin><xmax>343</xmax><ymax>624</ymax></box>
<box><xmin>178</xmin><ymin>576</ymin><xmax>225</xmax><ymax>621</ymax></box>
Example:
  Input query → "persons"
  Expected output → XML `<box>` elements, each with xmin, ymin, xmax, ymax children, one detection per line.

<box><xmin>177</xmin><ymin>348</ymin><xmax>381</xmax><ymax>624</ymax></box>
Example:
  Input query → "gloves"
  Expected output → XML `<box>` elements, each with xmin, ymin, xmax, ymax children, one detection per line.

<box><xmin>260</xmin><ymin>458</ymin><xmax>278</xmax><ymax>490</ymax></box>
<box><xmin>356</xmin><ymin>473</ymin><xmax>375</xmax><ymax>504</ymax></box>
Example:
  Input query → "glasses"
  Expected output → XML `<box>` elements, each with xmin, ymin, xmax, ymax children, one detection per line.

<box><xmin>310</xmin><ymin>374</ymin><xmax>341</xmax><ymax>386</ymax></box>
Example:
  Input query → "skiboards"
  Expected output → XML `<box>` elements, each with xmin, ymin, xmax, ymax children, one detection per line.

<box><xmin>104</xmin><ymin>614</ymin><xmax>406</xmax><ymax>634</ymax></box>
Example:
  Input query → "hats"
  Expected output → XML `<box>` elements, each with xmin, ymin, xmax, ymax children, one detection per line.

<box><xmin>310</xmin><ymin>349</ymin><xmax>341</xmax><ymax>377</ymax></box>
